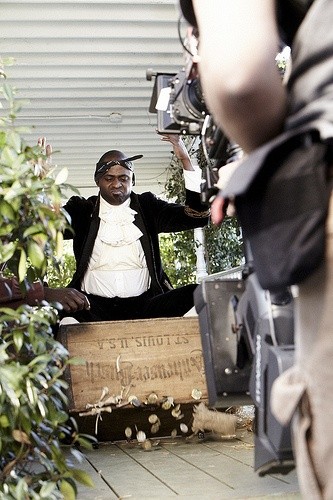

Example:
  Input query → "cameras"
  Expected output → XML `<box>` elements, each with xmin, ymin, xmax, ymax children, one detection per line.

<box><xmin>181</xmin><ymin>77</ymin><xmax>232</xmax><ymax>168</ymax></box>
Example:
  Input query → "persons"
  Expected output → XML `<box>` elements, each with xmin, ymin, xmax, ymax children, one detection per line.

<box><xmin>36</xmin><ymin>128</ymin><xmax>206</xmax><ymax>319</ymax></box>
<box><xmin>0</xmin><ymin>275</ymin><xmax>90</xmax><ymax>313</ymax></box>
<box><xmin>180</xmin><ymin>0</ymin><xmax>333</xmax><ymax>499</ymax></box>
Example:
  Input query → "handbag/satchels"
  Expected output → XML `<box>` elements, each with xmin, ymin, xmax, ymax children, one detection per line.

<box><xmin>232</xmin><ymin>128</ymin><xmax>333</xmax><ymax>292</ymax></box>
